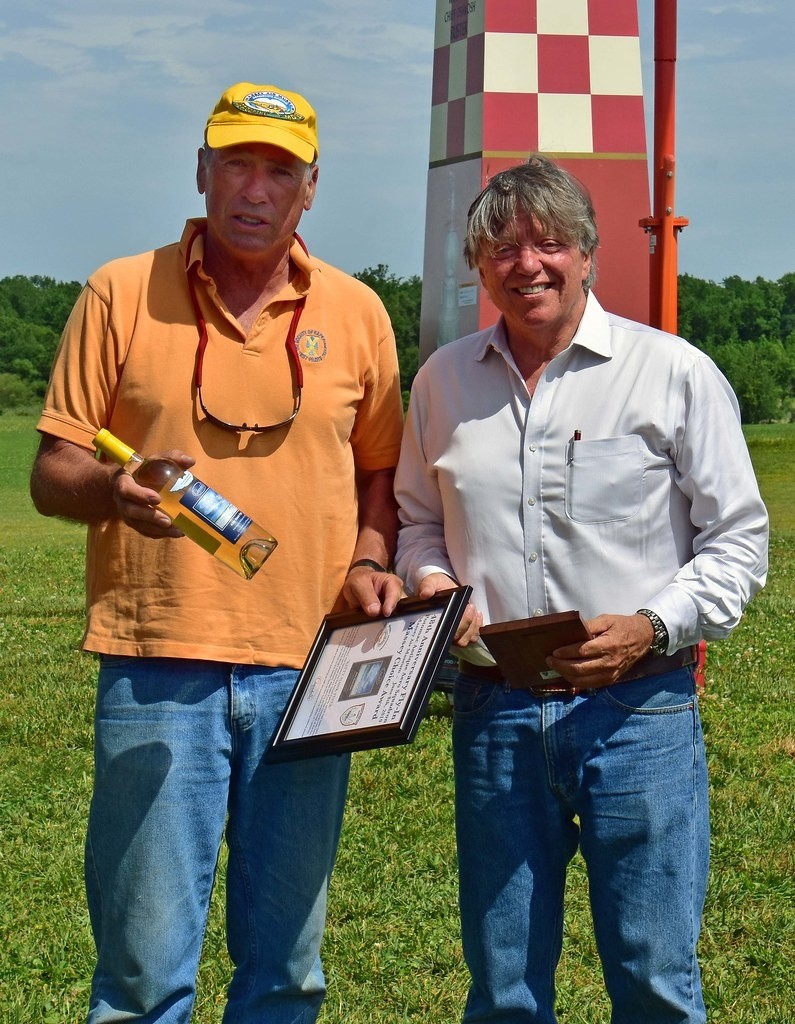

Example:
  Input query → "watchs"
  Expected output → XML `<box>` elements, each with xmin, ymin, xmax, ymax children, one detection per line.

<box><xmin>639</xmin><ymin>610</ymin><xmax>668</xmax><ymax>657</ymax></box>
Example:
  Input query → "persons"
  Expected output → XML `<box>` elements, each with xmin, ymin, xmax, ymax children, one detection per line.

<box><xmin>30</xmin><ymin>82</ymin><xmax>403</xmax><ymax>1024</ymax></box>
<box><xmin>393</xmin><ymin>157</ymin><xmax>768</xmax><ymax>1024</ymax></box>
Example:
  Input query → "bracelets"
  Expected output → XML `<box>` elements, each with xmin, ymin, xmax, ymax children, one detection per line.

<box><xmin>348</xmin><ymin>559</ymin><xmax>387</xmax><ymax>573</ymax></box>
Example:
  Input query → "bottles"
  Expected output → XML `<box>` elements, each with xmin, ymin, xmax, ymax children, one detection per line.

<box><xmin>91</xmin><ymin>427</ymin><xmax>278</xmax><ymax>581</ymax></box>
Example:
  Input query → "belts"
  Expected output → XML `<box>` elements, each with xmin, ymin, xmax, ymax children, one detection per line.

<box><xmin>459</xmin><ymin>642</ymin><xmax>697</xmax><ymax>696</ymax></box>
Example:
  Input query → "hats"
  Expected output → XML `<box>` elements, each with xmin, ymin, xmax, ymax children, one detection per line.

<box><xmin>203</xmin><ymin>82</ymin><xmax>318</xmax><ymax>163</ymax></box>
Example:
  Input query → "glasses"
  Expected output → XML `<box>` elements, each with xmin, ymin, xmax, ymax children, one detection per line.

<box><xmin>199</xmin><ymin>385</ymin><xmax>301</xmax><ymax>432</ymax></box>
